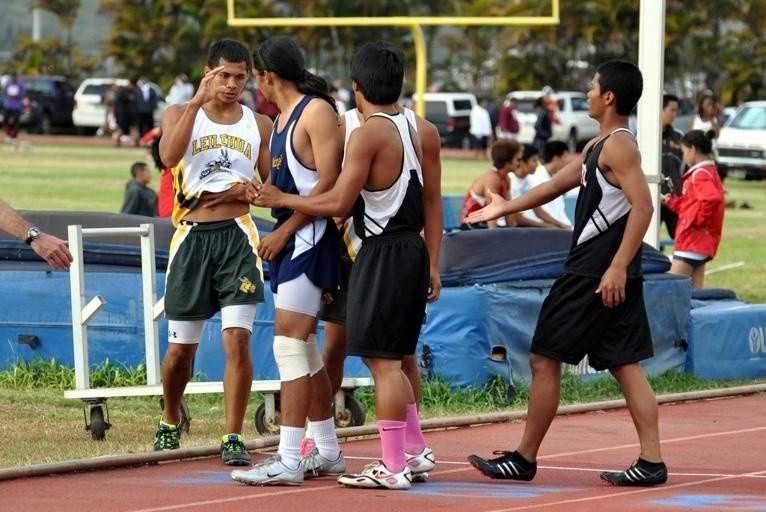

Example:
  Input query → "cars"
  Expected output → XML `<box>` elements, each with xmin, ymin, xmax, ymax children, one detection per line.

<box><xmin>712</xmin><ymin>100</ymin><xmax>766</xmax><ymax>180</ymax></box>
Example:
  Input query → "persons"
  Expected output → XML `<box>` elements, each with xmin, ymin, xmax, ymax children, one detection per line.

<box><xmin>2</xmin><ymin>70</ymin><xmax>25</xmax><ymax>142</ymax></box>
<box><xmin>153</xmin><ymin>38</ymin><xmax>274</xmax><ymax>465</ymax></box>
<box><xmin>98</xmin><ymin>65</ymin><xmax>192</xmax><ymax>150</ymax></box>
<box><xmin>459</xmin><ymin>84</ymin><xmax>574</xmax><ymax>232</ymax></box>
<box><xmin>462</xmin><ymin>60</ymin><xmax>668</xmax><ymax>486</ymax></box>
<box><xmin>236</xmin><ymin>35</ymin><xmax>445</xmax><ymax>488</ymax></box>
<box><xmin>661</xmin><ymin>94</ymin><xmax>728</xmax><ymax>289</ymax></box>
<box><xmin>120</xmin><ymin>136</ymin><xmax>174</xmax><ymax>218</ymax></box>
<box><xmin>0</xmin><ymin>202</ymin><xmax>74</xmax><ymax>272</ymax></box>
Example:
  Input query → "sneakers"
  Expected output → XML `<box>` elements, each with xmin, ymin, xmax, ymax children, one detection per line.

<box><xmin>467</xmin><ymin>451</ymin><xmax>537</xmax><ymax>481</ymax></box>
<box><xmin>155</xmin><ymin>412</ymin><xmax>182</xmax><ymax>449</ymax></box>
<box><xmin>600</xmin><ymin>460</ymin><xmax>667</xmax><ymax>485</ymax></box>
<box><xmin>220</xmin><ymin>432</ymin><xmax>435</xmax><ymax>489</ymax></box>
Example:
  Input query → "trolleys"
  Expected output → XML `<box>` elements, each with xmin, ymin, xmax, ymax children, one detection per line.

<box><xmin>59</xmin><ymin>222</ymin><xmax>375</xmax><ymax>438</ymax></box>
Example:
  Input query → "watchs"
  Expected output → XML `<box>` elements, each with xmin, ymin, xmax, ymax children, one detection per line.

<box><xmin>25</xmin><ymin>227</ymin><xmax>41</xmax><ymax>246</ymax></box>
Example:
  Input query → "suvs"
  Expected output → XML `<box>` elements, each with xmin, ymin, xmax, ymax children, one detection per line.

<box><xmin>495</xmin><ymin>90</ymin><xmax>602</xmax><ymax>154</ymax></box>
<box><xmin>408</xmin><ymin>90</ymin><xmax>480</xmax><ymax>150</ymax></box>
<box><xmin>70</xmin><ymin>77</ymin><xmax>166</xmax><ymax>134</ymax></box>
<box><xmin>0</xmin><ymin>73</ymin><xmax>99</xmax><ymax>134</ymax></box>
